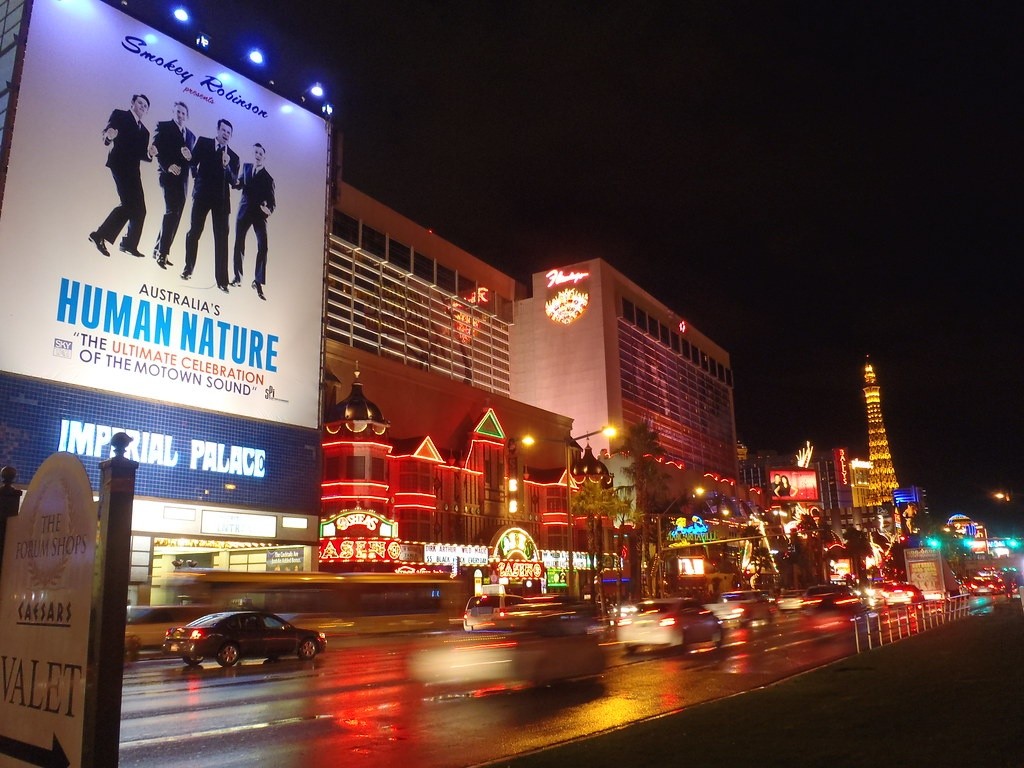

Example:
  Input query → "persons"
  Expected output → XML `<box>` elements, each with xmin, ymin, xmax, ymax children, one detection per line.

<box><xmin>708</xmin><ymin>580</ymin><xmax>716</xmax><ymax>595</ymax></box>
<box><xmin>771</xmin><ymin>474</ymin><xmax>798</xmax><ymax>497</ymax></box>
<box><xmin>1004</xmin><ymin>571</ymin><xmax>1013</xmax><ymax>602</ymax></box>
<box><xmin>86</xmin><ymin>93</ymin><xmax>277</xmax><ymax>302</ymax></box>
<box><xmin>1015</xmin><ymin>572</ymin><xmax>1024</xmax><ymax>612</ymax></box>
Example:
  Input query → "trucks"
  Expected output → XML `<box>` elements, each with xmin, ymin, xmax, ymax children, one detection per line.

<box><xmin>904</xmin><ymin>548</ymin><xmax>961</xmax><ymax>607</ymax></box>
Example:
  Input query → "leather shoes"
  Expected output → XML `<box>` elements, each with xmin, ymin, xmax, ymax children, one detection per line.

<box><xmin>153</xmin><ymin>251</ymin><xmax>173</xmax><ymax>266</ymax></box>
<box><xmin>120</xmin><ymin>241</ymin><xmax>144</xmax><ymax>257</ymax></box>
<box><xmin>229</xmin><ymin>282</ymin><xmax>241</xmax><ymax>287</ymax></box>
<box><xmin>252</xmin><ymin>280</ymin><xmax>266</xmax><ymax>300</ymax></box>
<box><xmin>181</xmin><ymin>274</ymin><xmax>191</xmax><ymax>280</ymax></box>
<box><xmin>218</xmin><ymin>285</ymin><xmax>229</xmax><ymax>293</ymax></box>
<box><xmin>88</xmin><ymin>231</ymin><xmax>110</xmax><ymax>256</ymax></box>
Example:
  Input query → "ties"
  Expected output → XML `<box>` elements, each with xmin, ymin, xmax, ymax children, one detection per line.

<box><xmin>217</xmin><ymin>144</ymin><xmax>222</xmax><ymax>152</ymax></box>
<box><xmin>253</xmin><ymin>168</ymin><xmax>256</xmax><ymax>178</ymax></box>
<box><xmin>138</xmin><ymin>120</ymin><xmax>142</xmax><ymax>130</ymax></box>
<box><xmin>182</xmin><ymin>127</ymin><xmax>186</xmax><ymax>142</ymax></box>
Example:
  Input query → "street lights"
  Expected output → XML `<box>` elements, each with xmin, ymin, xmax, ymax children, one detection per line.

<box><xmin>563</xmin><ymin>425</ymin><xmax>617</xmax><ymax>604</ymax></box>
<box><xmin>656</xmin><ymin>484</ymin><xmax>704</xmax><ymax>599</ymax></box>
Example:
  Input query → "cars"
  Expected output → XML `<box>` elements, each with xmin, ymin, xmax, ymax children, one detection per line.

<box><xmin>968</xmin><ymin>576</ymin><xmax>1005</xmax><ymax>597</ymax></box>
<box><xmin>161</xmin><ymin>610</ymin><xmax>327</xmax><ymax>668</ymax></box>
<box><xmin>778</xmin><ymin>581</ymin><xmax>925</xmax><ymax>614</ymax></box>
<box><xmin>702</xmin><ymin>590</ymin><xmax>777</xmax><ymax>629</ymax></box>
<box><xmin>463</xmin><ymin>593</ymin><xmax>527</xmax><ymax>634</ymax></box>
<box><xmin>622</xmin><ymin>597</ymin><xmax>724</xmax><ymax>655</ymax></box>
